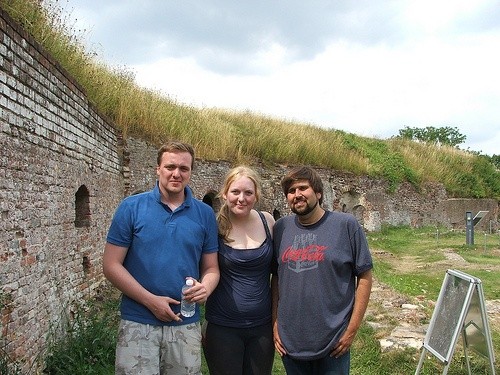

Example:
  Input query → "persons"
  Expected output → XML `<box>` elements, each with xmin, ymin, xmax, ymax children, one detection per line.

<box><xmin>103</xmin><ymin>142</ymin><xmax>220</xmax><ymax>375</ymax></box>
<box><xmin>271</xmin><ymin>166</ymin><xmax>373</xmax><ymax>375</ymax></box>
<box><xmin>198</xmin><ymin>166</ymin><xmax>276</xmax><ymax>375</ymax></box>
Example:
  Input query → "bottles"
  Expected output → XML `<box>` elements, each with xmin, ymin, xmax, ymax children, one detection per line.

<box><xmin>181</xmin><ymin>280</ymin><xmax>195</xmax><ymax>317</ymax></box>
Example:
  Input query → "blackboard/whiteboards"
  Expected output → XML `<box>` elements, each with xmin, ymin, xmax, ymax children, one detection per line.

<box><xmin>414</xmin><ymin>267</ymin><xmax>496</xmax><ymax>375</ymax></box>
